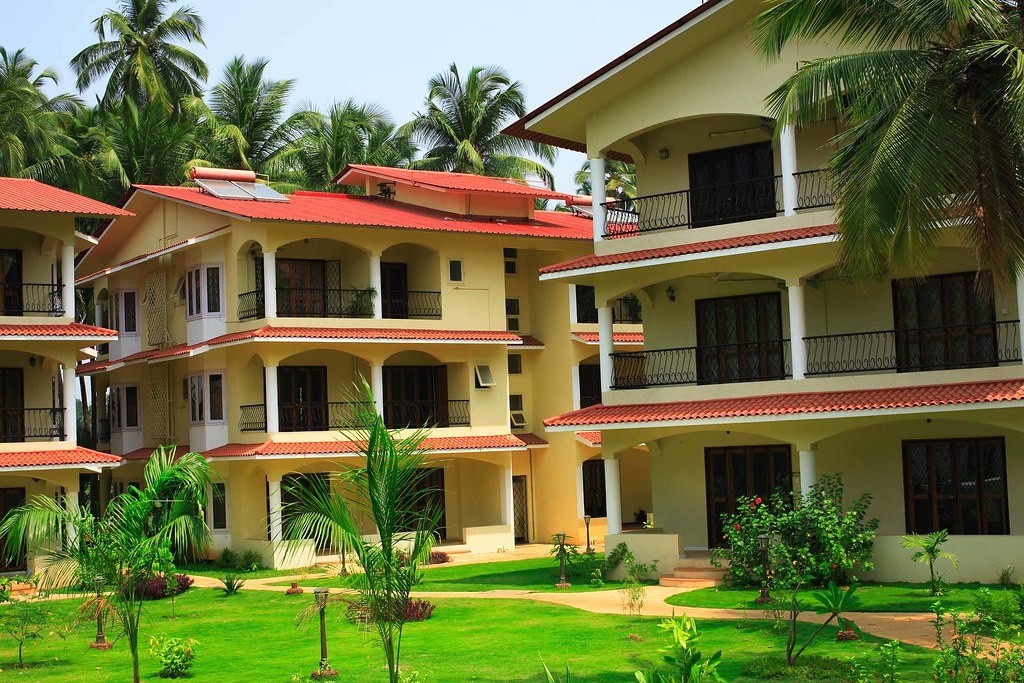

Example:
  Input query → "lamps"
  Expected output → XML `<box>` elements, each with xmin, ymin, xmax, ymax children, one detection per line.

<box><xmin>666</xmin><ymin>286</ymin><xmax>675</xmax><ymax>302</ymax></box>
<box><xmin>29</xmin><ymin>356</ymin><xmax>36</xmax><ymax>366</ymax></box>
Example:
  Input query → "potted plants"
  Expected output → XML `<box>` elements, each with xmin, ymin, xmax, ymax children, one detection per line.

<box><xmin>351</xmin><ymin>287</ymin><xmax>376</xmax><ymax>318</ymax></box>
